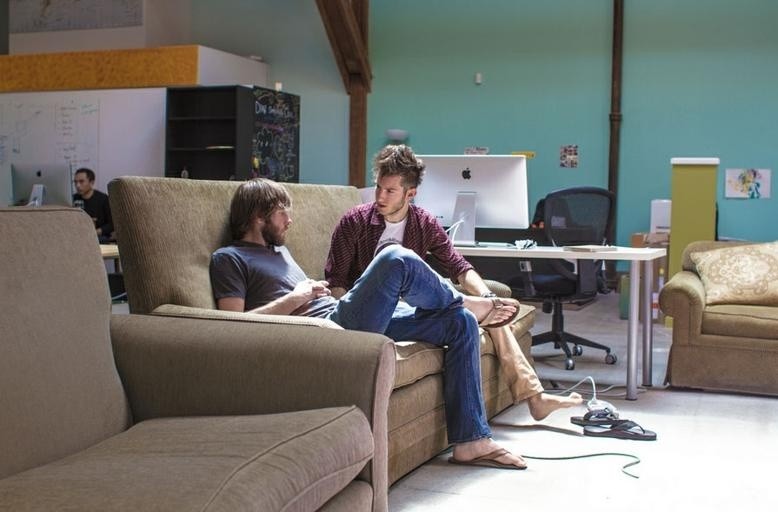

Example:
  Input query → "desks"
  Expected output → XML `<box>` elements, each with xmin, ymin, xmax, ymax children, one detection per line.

<box><xmin>425</xmin><ymin>240</ymin><xmax>668</xmax><ymax>401</ymax></box>
<box><xmin>100</xmin><ymin>244</ymin><xmax>119</xmax><ymax>258</ymax></box>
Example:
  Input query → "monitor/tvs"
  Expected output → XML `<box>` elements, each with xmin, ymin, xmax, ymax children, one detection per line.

<box><xmin>414</xmin><ymin>154</ymin><xmax>530</xmax><ymax>246</ymax></box>
<box><xmin>11</xmin><ymin>160</ymin><xmax>73</xmax><ymax>208</ymax></box>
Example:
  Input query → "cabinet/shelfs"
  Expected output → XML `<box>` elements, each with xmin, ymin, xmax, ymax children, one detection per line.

<box><xmin>164</xmin><ymin>84</ymin><xmax>255</xmax><ymax>181</ymax></box>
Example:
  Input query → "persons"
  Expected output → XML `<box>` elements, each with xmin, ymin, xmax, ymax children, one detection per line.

<box><xmin>326</xmin><ymin>144</ymin><xmax>583</xmax><ymax>421</ymax></box>
<box><xmin>210</xmin><ymin>178</ymin><xmax>528</xmax><ymax>471</ymax></box>
<box><xmin>69</xmin><ymin>167</ymin><xmax>116</xmax><ymax>242</ymax></box>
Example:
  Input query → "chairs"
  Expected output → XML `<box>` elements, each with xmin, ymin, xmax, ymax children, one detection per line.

<box><xmin>658</xmin><ymin>241</ymin><xmax>778</xmax><ymax>397</ymax></box>
<box><xmin>512</xmin><ymin>186</ymin><xmax>617</xmax><ymax>370</ymax></box>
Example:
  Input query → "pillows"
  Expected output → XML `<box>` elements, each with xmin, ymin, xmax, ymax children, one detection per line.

<box><xmin>690</xmin><ymin>243</ymin><xmax>778</xmax><ymax>304</ymax></box>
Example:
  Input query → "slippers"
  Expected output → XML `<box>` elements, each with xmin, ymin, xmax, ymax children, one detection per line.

<box><xmin>570</xmin><ymin>406</ymin><xmax>619</xmax><ymax>425</ymax></box>
<box><xmin>477</xmin><ymin>297</ymin><xmax>520</xmax><ymax>328</ymax></box>
<box><xmin>584</xmin><ymin>420</ymin><xmax>656</xmax><ymax>440</ymax></box>
<box><xmin>447</xmin><ymin>448</ymin><xmax>527</xmax><ymax>469</ymax></box>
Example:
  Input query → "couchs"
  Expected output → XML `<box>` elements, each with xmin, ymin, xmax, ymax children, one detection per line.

<box><xmin>0</xmin><ymin>206</ymin><xmax>396</xmax><ymax>512</ymax></box>
<box><xmin>107</xmin><ymin>175</ymin><xmax>536</xmax><ymax>490</ymax></box>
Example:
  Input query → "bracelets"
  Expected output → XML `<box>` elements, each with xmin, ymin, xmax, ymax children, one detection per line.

<box><xmin>478</xmin><ymin>289</ymin><xmax>497</xmax><ymax>298</ymax></box>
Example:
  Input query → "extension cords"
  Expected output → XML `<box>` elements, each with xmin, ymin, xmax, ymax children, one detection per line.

<box><xmin>587</xmin><ymin>399</ymin><xmax>619</xmax><ymax>419</ymax></box>
